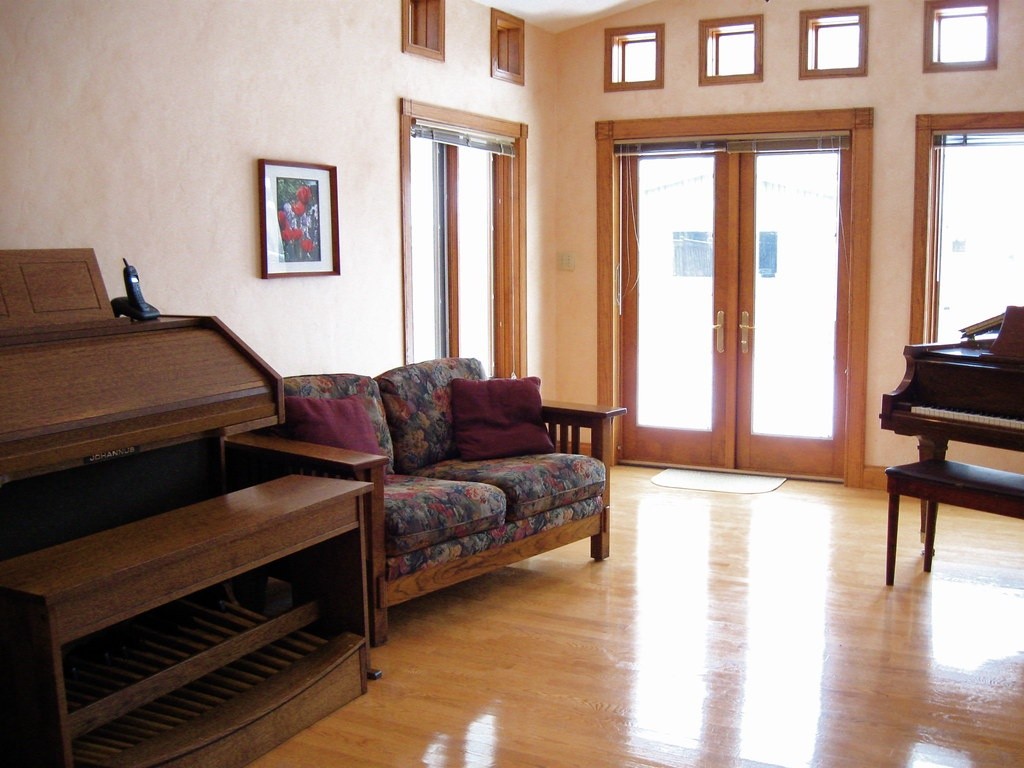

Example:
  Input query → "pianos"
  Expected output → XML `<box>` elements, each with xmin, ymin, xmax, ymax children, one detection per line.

<box><xmin>1</xmin><ymin>245</ymin><xmax>378</xmax><ymax>768</ymax></box>
<box><xmin>878</xmin><ymin>305</ymin><xmax>1024</xmax><ymax>542</ymax></box>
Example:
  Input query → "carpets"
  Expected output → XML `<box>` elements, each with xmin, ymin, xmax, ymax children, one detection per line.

<box><xmin>651</xmin><ymin>468</ymin><xmax>787</xmax><ymax>493</ymax></box>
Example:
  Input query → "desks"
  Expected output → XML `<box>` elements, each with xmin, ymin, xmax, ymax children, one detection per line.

<box><xmin>887</xmin><ymin>459</ymin><xmax>1024</xmax><ymax>586</ymax></box>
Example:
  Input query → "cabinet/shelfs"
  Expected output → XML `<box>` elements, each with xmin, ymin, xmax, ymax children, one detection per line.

<box><xmin>258</xmin><ymin>158</ymin><xmax>341</xmax><ymax>279</ymax></box>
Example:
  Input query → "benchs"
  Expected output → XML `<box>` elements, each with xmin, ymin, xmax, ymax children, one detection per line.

<box><xmin>0</xmin><ymin>474</ymin><xmax>374</xmax><ymax>767</ymax></box>
<box><xmin>220</xmin><ymin>358</ymin><xmax>627</xmax><ymax>647</ymax></box>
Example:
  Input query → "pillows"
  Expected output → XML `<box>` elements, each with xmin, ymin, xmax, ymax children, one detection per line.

<box><xmin>285</xmin><ymin>395</ymin><xmax>381</xmax><ymax>479</ymax></box>
<box><xmin>453</xmin><ymin>377</ymin><xmax>553</xmax><ymax>460</ymax></box>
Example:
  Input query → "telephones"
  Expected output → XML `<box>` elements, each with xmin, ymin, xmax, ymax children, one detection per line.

<box><xmin>110</xmin><ymin>257</ymin><xmax>161</xmax><ymax>321</ymax></box>
